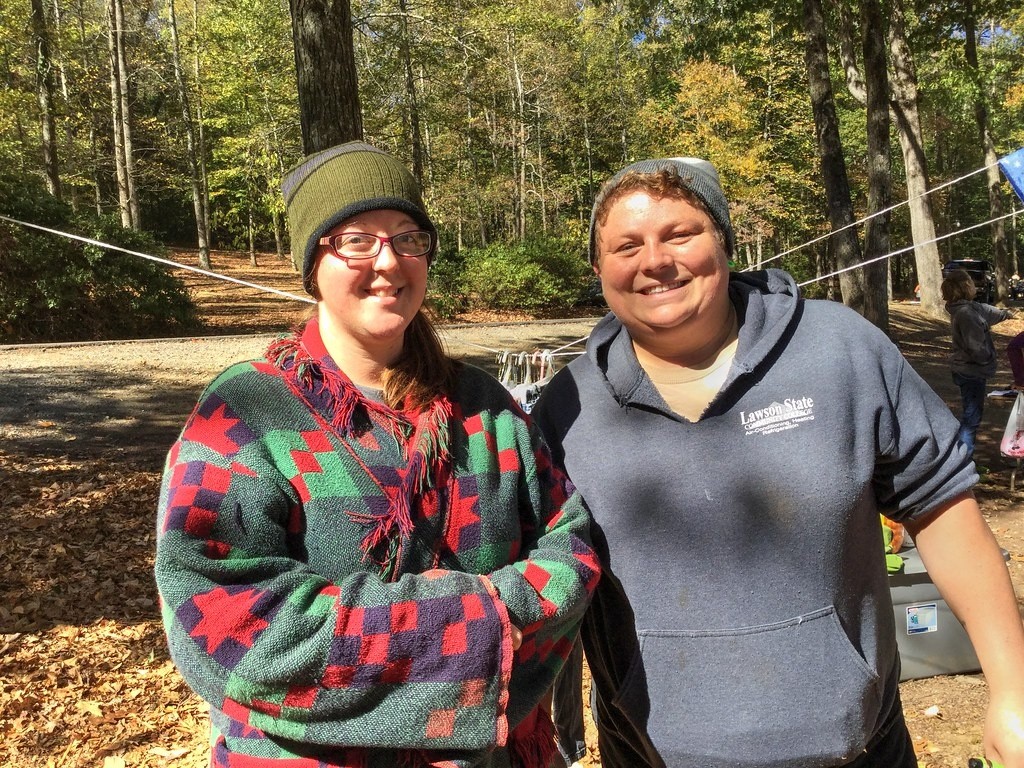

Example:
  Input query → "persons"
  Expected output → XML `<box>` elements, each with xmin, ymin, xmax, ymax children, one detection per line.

<box><xmin>1011</xmin><ymin>270</ymin><xmax>1020</xmax><ymax>301</ymax></box>
<box><xmin>941</xmin><ymin>269</ymin><xmax>1019</xmax><ymax>465</ymax></box>
<box><xmin>153</xmin><ymin>141</ymin><xmax>605</xmax><ymax>768</ymax></box>
<box><xmin>529</xmin><ymin>154</ymin><xmax>1024</xmax><ymax>768</ymax></box>
<box><xmin>1007</xmin><ymin>331</ymin><xmax>1024</xmax><ymax>391</ymax></box>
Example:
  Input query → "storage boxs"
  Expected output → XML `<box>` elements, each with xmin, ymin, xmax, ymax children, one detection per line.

<box><xmin>888</xmin><ymin>546</ymin><xmax>1011</xmax><ymax>681</ymax></box>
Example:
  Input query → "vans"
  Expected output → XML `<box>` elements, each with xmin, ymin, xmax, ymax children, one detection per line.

<box><xmin>941</xmin><ymin>258</ymin><xmax>995</xmax><ymax>304</ymax></box>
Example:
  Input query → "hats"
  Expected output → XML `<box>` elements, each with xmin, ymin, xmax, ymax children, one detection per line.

<box><xmin>585</xmin><ymin>157</ymin><xmax>733</xmax><ymax>268</ymax></box>
<box><xmin>280</xmin><ymin>138</ymin><xmax>438</xmax><ymax>300</ymax></box>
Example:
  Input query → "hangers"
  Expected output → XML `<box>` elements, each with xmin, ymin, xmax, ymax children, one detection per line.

<box><xmin>495</xmin><ymin>349</ymin><xmax>560</xmax><ymax>395</ymax></box>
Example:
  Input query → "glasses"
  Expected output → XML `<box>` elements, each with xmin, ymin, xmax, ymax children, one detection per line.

<box><xmin>318</xmin><ymin>228</ymin><xmax>432</xmax><ymax>259</ymax></box>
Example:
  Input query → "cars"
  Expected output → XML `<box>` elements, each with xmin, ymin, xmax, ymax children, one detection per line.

<box><xmin>1007</xmin><ymin>278</ymin><xmax>1024</xmax><ymax>302</ymax></box>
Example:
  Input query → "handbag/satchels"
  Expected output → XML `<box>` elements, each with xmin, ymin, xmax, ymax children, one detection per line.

<box><xmin>999</xmin><ymin>390</ymin><xmax>1024</xmax><ymax>458</ymax></box>
